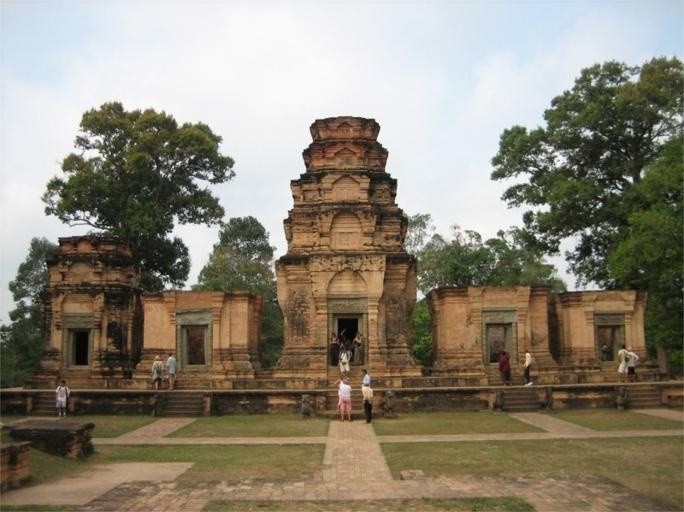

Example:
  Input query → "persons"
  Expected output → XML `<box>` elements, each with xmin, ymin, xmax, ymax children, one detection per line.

<box><xmin>151</xmin><ymin>352</ymin><xmax>177</xmax><ymax>391</ymax></box>
<box><xmin>330</xmin><ymin>331</ymin><xmax>374</xmax><ymax>424</ymax></box>
<box><xmin>499</xmin><ymin>349</ymin><xmax>534</xmax><ymax>386</ymax></box>
<box><xmin>55</xmin><ymin>380</ymin><xmax>71</xmax><ymax>418</ymax></box>
<box><xmin>617</xmin><ymin>344</ymin><xmax>639</xmax><ymax>384</ymax></box>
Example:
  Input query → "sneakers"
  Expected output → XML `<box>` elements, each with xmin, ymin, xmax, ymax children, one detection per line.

<box><xmin>524</xmin><ymin>381</ymin><xmax>533</xmax><ymax>386</ymax></box>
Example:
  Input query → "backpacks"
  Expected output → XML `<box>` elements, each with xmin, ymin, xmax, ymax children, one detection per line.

<box><xmin>156</xmin><ymin>366</ymin><xmax>162</xmax><ymax>374</ymax></box>
